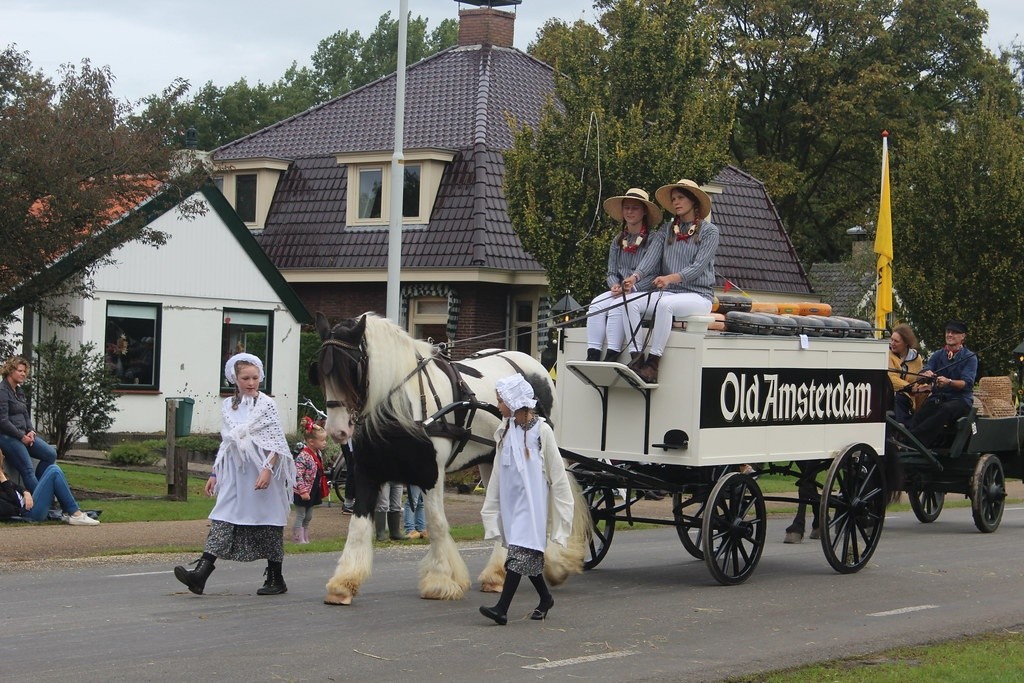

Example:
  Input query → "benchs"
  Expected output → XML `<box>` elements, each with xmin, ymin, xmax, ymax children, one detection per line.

<box><xmin>886</xmin><ymin>407</ymin><xmax>978</xmax><ymax>430</ymax></box>
<box><xmin>3</xmin><ymin>445</ymin><xmax>57</xmax><ymax>502</ymax></box>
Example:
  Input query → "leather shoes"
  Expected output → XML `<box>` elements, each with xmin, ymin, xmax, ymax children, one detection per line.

<box><xmin>479</xmin><ymin>606</ymin><xmax>508</xmax><ymax>625</ymax></box>
<box><xmin>531</xmin><ymin>594</ymin><xmax>554</xmax><ymax>620</ymax></box>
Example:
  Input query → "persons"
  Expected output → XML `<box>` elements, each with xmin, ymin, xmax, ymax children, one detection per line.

<box><xmin>0</xmin><ymin>356</ymin><xmax>58</xmax><ymax>506</ymax></box>
<box><xmin>586</xmin><ymin>188</ymin><xmax>664</xmax><ymax>362</ymax></box>
<box><xmin>290</xmin><ymin>417</ymin><xmax>328</xmax><ymax>544</ymax></box>
<box><xmin>174</xmin><ymin>353</ymin><xmax>296</xmax><ymax>595</ymax></box>
<box><xmin>621</xmin><ymin>178</ymin><xmax>719</xmax><ymax>383</ymax></box>
<box><xmin>887</xmin><ymin>322</ymin><xmax>930</xmax><ymax>443</ymax></box>
<box><xmin>404</xmin><ymin>483</ymin><xmax>429</xmax><ymax>539</ymax></box>
<box><xmin>375</xmin><ymin>481</ymin><xmax>410</xmax><ymax>540</ymax></box>
<box><xmin>902</xmin><ymin>321</ymin><xmax>977</xmax><ymax>450</ymax></box>
<box><xmin>476</xmin><ymin>374</ymin><xmax>574</xmax><ymax>625</ymax></box>
<box><xmin>0</xmin><ymin>451</ymin><xmax>100</xmax><ymax>526</ymax></box>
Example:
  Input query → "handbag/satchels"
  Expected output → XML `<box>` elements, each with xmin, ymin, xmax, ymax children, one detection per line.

<box><xmin>320</xmin><ymin>476</ymin><xmax>330</xmax><ymax>498</ymax></box>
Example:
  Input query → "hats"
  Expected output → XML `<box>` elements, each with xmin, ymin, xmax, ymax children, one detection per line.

<box><xmin>945</xmin><ymin>319</ymin><xmax>968</xmax><ymax>333</ymax></box>
<box><xmin>604</xmin><ymin>188</ymin><xmax>663</xmax><ymax>225</ymax></box>
<box><xmin>495</xmin><ymin>372</ymin><xmax>538</xmax><ymax>473</ymax></box>
<box><xmin>655</xmin><ymin>179</ymin><xmax>710</xmax><ymax>219</ymax></box>
<box><xmin>225</xmin><ymin>352</ymin><xmax>265</xmax><ymax>461</ymax></box>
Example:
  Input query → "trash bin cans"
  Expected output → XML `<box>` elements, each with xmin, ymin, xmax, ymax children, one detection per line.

<box><xmin>164</xmin><ymin>397</ymin><xmax>195</xmax><ymax>436</ymax></box>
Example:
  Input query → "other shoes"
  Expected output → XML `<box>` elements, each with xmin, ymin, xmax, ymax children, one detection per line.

<box><xmin>49</xmin><ymin>508</ymin><xmax>62</xmax><ymax>519</ymax></box>
<box><xmin>419</xmin><ymin>531</ymin><xmax>428</xmax><ymax>537</ymax></box>
<box><xmin>68</xmin><ymin>512</ymin><xmax>100</xmax><ymax>526</ymax></box>
<box><xmin>405</xmin><ymin>529</ymin><xmax>420</xmax><ymax>538</ymax></box>
<box><xmin>60</xmin><ymin>513</ymin><xmax>68</xmax><ymax>523</ymax></box>
<box><xmin>341</xmin><ymin>502</ymin><xmax>354</xmax><ymax>515</ymax></box>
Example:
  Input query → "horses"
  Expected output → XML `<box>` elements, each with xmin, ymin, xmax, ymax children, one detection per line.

<box><xmin>784</xmin><ymin>460</ymin><xmax>901</xmax><ymax>544</ymax></box>
<box><xmin>315</xmin><ymin>310</ymin><xmax>589</xmax><ymax>605</ymax></box>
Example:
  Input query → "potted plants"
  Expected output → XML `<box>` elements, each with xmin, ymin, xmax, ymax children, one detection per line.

<box><xmin>145</xmin><ymin>435</ymin><xmax>222</xmax><ymax>461</ymax></box>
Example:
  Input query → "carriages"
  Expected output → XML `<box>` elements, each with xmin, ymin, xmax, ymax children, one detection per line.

<box><xmin>783</xmin><ymin>367</ymin><xmax>1024</xmax><ymax>544</ymax></box>
<box><xmin>308</xmin><ymin>295</ymin><xmax>888</xmax><ymax>605</ymax></box>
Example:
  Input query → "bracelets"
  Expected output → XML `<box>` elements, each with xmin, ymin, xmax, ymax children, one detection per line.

<box><xmin>948</xmin><ymin>379</ymin><xmax>953</xmax><ymax>388</ymax></box>
<box><xmin>631</xmin><ymin>273</ymin><xmax>639</xmax><ymax>283</ymax></box>
<box><xmin>265</xmin><ymin>462</ymin><xmax>275</xmax><ymax>469</ymax></box>
<box><xmin>209</xmin><ymin>473</ymin><xmax>216</xmax><ymax>477</ymax></box>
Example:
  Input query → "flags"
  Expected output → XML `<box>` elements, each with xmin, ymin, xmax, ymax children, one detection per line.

<box><xmin>873</xmin><ymin>137</ymin><xmax>894</xmax><ymax>340</ymax></box>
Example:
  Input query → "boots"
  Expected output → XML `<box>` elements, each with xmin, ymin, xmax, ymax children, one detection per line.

<box><xmin>374</xmin><ymin>511</ymin><xmax>391</xmax><ymax>543</ymax></box>
<box><xmin>174</xmin><ymin>557</ymin><xmax>215</xmax><ymax>595</ymax></box>
<box><xmin>635</xmin><ymin>353</ymin><xmax>660</xmax><ymax>383</ymax></box>
<box><xmin>603</xmin><ymin>349</ymin><xmax>620</xmax><ymax>362</ymax></box>
<box><xmin>292</xmin><ymin>527</ymin><xmax>310</xmax><ymax>545</ymax></box>
<box><xmin>256</xmin><ymin>561</ymin><xmax>287</xmax><ymax>595</ymax></box>
<box><xmin>626</xmin><ymin>351</ymin><xmax>645</xmax><ymax>371</ymax></box>
<box><xmin>387</xmin><ymin>511</ymin><xmax>407</xmax><ymax>540</ymax></box>
<box><xmin>586</xmin><ymin>348</ymin><xmax>602</xmax><ymax>361</ymax></box>
